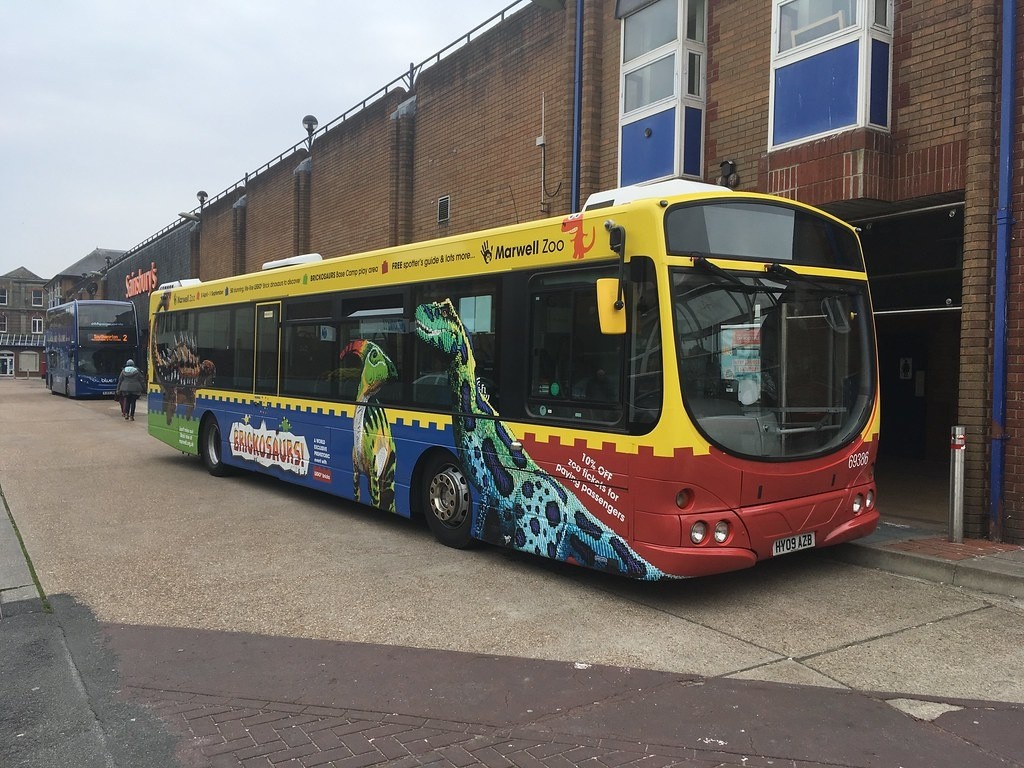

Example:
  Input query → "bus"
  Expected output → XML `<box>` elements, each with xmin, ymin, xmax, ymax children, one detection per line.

<box><xmin>44</xmin><ymin>297</ymin><xmax>141</xmax><ymax>400</ymax></box>
<box><xmin>146</xmin><ymin>180</ymin><xmax>883</xmax><ymax>585</ymax></box>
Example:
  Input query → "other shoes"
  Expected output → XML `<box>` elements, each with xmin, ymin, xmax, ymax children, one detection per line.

<box><xmin>130</xmin><ymin>416</ymin><xmax>134</xmax><ymax>421</ymax></box>
<box><xmin>121</xmin><ymin>413</ymin><xmax>130</xmax><ymax>417</ymax></box>
<box><xmin>124</xmin><ymin>413</ymin><xmax>129</xmax><ymax>419</ymax></box>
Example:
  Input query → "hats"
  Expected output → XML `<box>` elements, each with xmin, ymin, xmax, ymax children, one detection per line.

<box><xmin>126</xmin><ymin>359</ymin><xmax>133</xmax><ymax>365</ymax></box>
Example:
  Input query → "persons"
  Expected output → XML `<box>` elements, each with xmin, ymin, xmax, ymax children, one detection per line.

<box><xmin>116</xmin><ymin>359</ymin><xmax>146</xmax><ymax>421</ymax></box>
<box><xmin>116</xmin><ymin>365</ymin><xmax>134</xmax><ymax>418</ymax></box>
<box><xmin>584</xmin><ymin>366</ymin><xmax>622</xmax><ymax>424</ymax></box>
